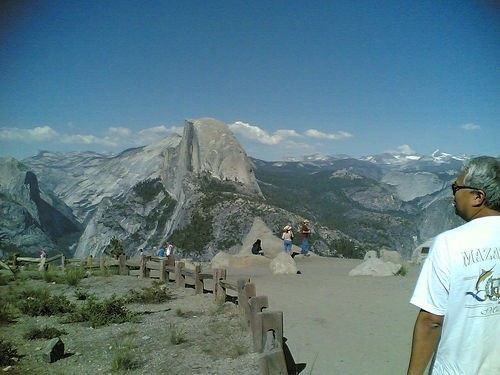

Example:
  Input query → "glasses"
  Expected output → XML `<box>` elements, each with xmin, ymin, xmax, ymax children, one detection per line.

<box><xmin>451</xmin><ymin>181</ymin><xmax>478</xmax><ymax>195</ymax></box>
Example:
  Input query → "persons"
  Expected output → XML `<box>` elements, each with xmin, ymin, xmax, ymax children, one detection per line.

<box><xmin>251</xmin><ymin>238</ymin><xmax>266</xmax><ymax>257</ymax></box>
<box><xmin>40</xmin><ymin>248</ymin><xmax>49</xmax><ymax>273</ymax></box>
<box><xmin>152</xmin><ymin>246</ymin><xmax>157</xmax><ymax>256</ymax></box>
<box><xmin>404</xmin><ymin>155</ymin><xmax>500</xmax><ymax>375</ymax></box>
<box><xmin>281</xmin><ymin>225</ymin><xmax>294</xmax><ymax>253</ymax></box>
<box><xmin>139</xmin><ymin>248</ymin><xmax>144</xmax><ymax>258</ymax></box>
<box><xmin>158</xmin><ymin>242</ymin><xmax>164</xmax><ymax>256</ymax></box>
<box><xmin>300</xmin><ymin>219</ymin><xmax>312</xmax><ymax>256</ymax></box>
<box><xmin>166</xmin><ymin>241</ymin><xmax>174</xmax><ymax>257</ymax></box>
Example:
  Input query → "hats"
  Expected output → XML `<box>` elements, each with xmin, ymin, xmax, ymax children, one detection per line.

<box><xmin>284</xmin><ymin>226</ymin><xmax>292</xmax><ymax>230</ymax></box>
<box><xmin>304</xmin><ymin>219</ymin><xmax>309</xmax><ymax>223</ymax></box>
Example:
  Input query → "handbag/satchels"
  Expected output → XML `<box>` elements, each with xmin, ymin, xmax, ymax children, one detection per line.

<box><xmin>290</xmin><ymin>231</ymin><xmax>293</xmax><ymax>240</ymax></box>
